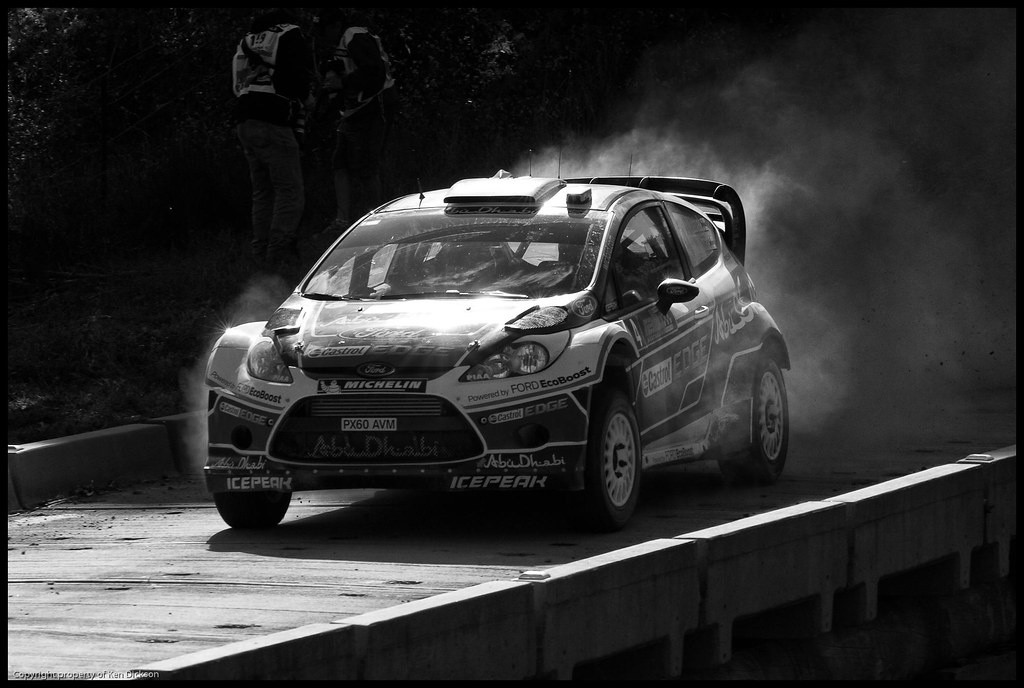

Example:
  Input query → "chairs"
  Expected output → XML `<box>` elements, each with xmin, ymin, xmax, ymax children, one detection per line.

<box><xmin>431</xmin><ymin>240</ymin><xmax>583</xmax><ymax>300</ymax></box>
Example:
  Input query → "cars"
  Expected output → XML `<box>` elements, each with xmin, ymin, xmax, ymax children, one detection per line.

<box><xmin>200</xmin><ymin>174</ymin><xmax>796</xmax><ymax>529</ymax></box>
